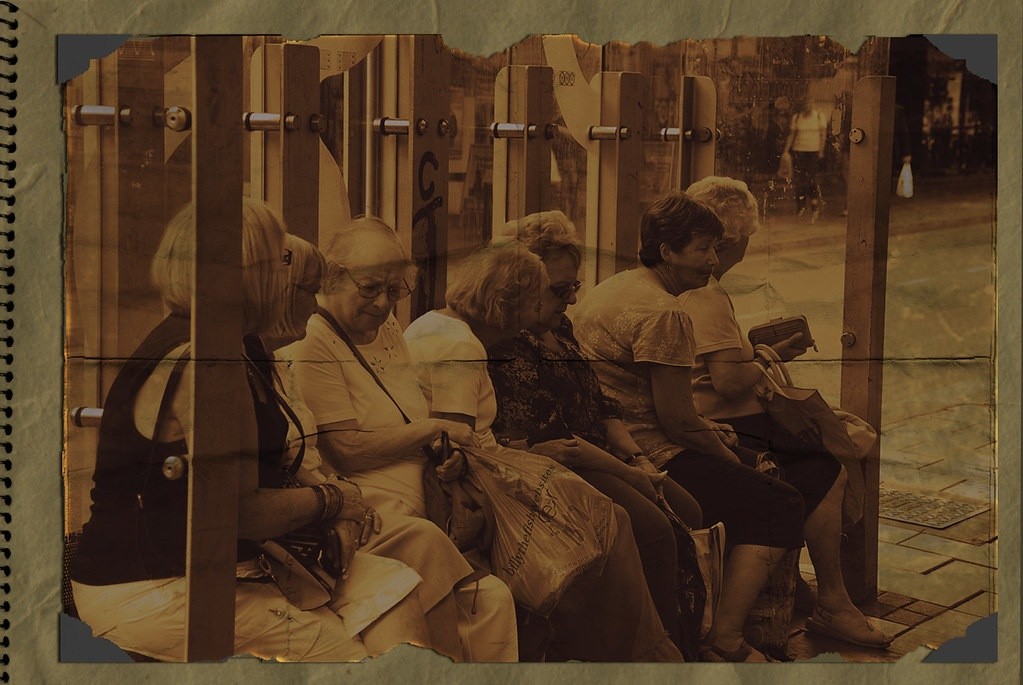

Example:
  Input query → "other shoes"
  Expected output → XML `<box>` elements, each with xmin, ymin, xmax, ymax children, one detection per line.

<box><xmin>810</xmin><ymin>209</ymin><xmax>820</xmax><ymax>225</ymax></box>
<box><xmin>796</xmin><ymin>206</ymin><xmax>806</xmax><ymax>216</ymax></box>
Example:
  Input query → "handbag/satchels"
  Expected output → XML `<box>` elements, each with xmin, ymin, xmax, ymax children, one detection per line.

<box><xmin>657</xmin><ymin>494</ymin><xmax>707</xmax><ymax>662</ymax></box>
<box><xmin>461</xmin><ymin>445</ymin><xmax>619</xmax><ymax>620</ymax></box>
<box><xmin>896</xmin><ymin>163</ymin><xmax>913</xmax><ymax>198</ymax></box>
<box><xmin>423</xmin><ymin>431</ymin><xmax>495</xmax><ymax>555</ymax></box>
<box><xmin>776</xmin><ymin>152</ymin><xmax>793</xmax><ymax>179</ymax></box>
<box><xmin>751</xmin><ymin>360</ymin><xmax>866</xmax><ymax>497</ymax></box>
<box><xmin>688</xmin><ymin>522</ymin><xmax>726</xmax><ymax>635</ymax></box>
<box><xmin>256</xmin><ymin>474</ymin><xmax>342</xmax><ymax>612</ymax></box>
<box><xmin>753</xmin><ymin>343</ymin><xmax>878</xmax><ymax>459</ymax></box>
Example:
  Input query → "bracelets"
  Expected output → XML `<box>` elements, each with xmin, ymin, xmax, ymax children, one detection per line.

<box><xmin>624</xmin><ymin>453</ymin><xmax>643</xmax><ymax>465</ymax></box>
<box><xmin>310</xmin><ymin>483</ymin><xmax>344</xmax><ymax>521</ymax></box>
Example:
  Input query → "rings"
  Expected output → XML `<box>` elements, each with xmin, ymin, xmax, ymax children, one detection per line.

<box><xmin>365</xmin><ymin>514</ymin><xmax>373</xmax><ymax>520</ymax></box>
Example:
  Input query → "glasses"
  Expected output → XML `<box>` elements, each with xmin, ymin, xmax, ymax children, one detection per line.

<box><xmin>549</xmin><ymin>280</ymin><xmax>582</xmax><ymax>299</ymax></box>
<box><xmin>281</xmin><ymin>247</ymin><xmax>293</xmax><ymax>265</ymax></box>
<box><xmin>344</xmin><ymin>267</ymin><xmax>412</xmax><ymax>301</ymax></box>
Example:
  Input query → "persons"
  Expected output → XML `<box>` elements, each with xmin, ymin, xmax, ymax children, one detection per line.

<box><xmin>72</xmin><ymin>196</ymin><xmax>424</xmax><ymax>665</ymax></box>
<box><xmin>784</xmin><ymin>96</ymin><xmax>827</xmax><ymax>213</ymax></box>
<box><xmin>892</xmin><ymin>103</ymin><xmax>911</xmax><ymax>169</ymax></box>
<box><xmin>290</xmin><ymin>215</ymin><xmax>519</xmax><ymax>663</ymax></box>
<box><xmin>679</xmin><ymin>176</ymin><xmax>892</xmax><ymax>650</ymax></box>
<box><xmin>570</xmin><ymin>194</ymin><xmax>806</xmax><ymax>664</ymax></box>
<box><xmin>255</xmin><ymin>234</ymin><xmax>474</xmax><ymax>664</ymax></box>
<box><xmin>401</xmin><ymin>211</ymin><xmax>703</xmax><ymax>664</ymax></box>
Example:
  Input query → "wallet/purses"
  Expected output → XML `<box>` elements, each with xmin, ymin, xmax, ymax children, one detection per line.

<box><xmin>748</xmin><ymin>315</ymin><xmax>819</xmax><ymax>352</ymax></box>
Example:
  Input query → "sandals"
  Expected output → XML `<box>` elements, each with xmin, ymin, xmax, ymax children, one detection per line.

<box><xmin>794</xmin><ymin>579</ymin><xmax>820</xmax><ymax>611</ymax></box>
<box><xmin>704</xmin><ymin>638</ymin><xmax>782</xmax><ymax>663</ymax></box>
<box><xmin>805</xmin><ymin>605</ymin><xmax>891</xmax><ymax>648</ymax></box>
